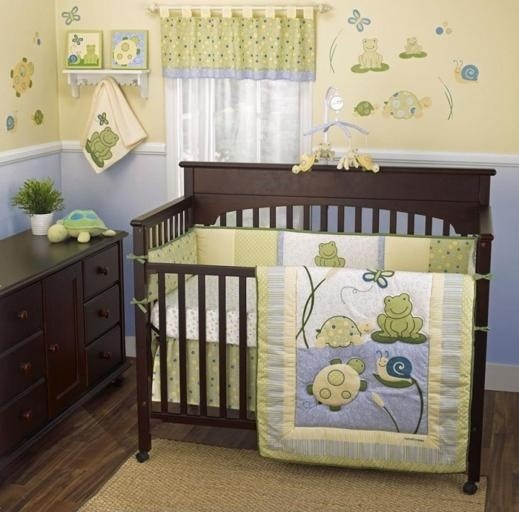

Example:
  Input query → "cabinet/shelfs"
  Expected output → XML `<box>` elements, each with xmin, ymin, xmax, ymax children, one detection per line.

<box><xmin>0</xmin><ymin>227</ymin><xmax>130</xmax><ymax>474</ymax></box>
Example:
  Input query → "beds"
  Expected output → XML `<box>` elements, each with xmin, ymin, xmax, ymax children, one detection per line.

<box><xmin>131</xmin><ymin>159</ymin><xmax>496</xmax><ymax>496</ymax></box>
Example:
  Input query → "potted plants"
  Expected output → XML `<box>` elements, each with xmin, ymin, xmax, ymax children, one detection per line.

<box><xmin>7</xmin><ymin>176</ymin><xmax>67</xmax><ymax>235</ymax></box>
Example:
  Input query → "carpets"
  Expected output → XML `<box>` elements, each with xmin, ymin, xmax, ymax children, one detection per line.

<box><xmin>77</xmin><ymin>436</ymin><xmax>490</xmax><ymax>512</ymax></box>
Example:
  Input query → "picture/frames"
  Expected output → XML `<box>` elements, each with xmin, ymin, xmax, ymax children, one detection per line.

<box><xmin>63</xmin><ymin>28</ymin><xmax>149</xmax><ymax>71</ymax></box>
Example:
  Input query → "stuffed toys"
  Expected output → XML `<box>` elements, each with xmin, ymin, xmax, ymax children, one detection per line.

<box><xmin>291</xmin><ymin>88</ymin><xmax>379</xmax><ymax>174</ymax></box>
<box><xmin>46</xmin><ymin>209</ymin><xmax>115</xmax><ymax>244</ymax></box>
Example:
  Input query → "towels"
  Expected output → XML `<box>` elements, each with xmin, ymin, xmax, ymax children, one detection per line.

<box><xmin>80</xmin><ymin>77</ymin><xmax>148</xmax><ymax>176</ymax></box>
